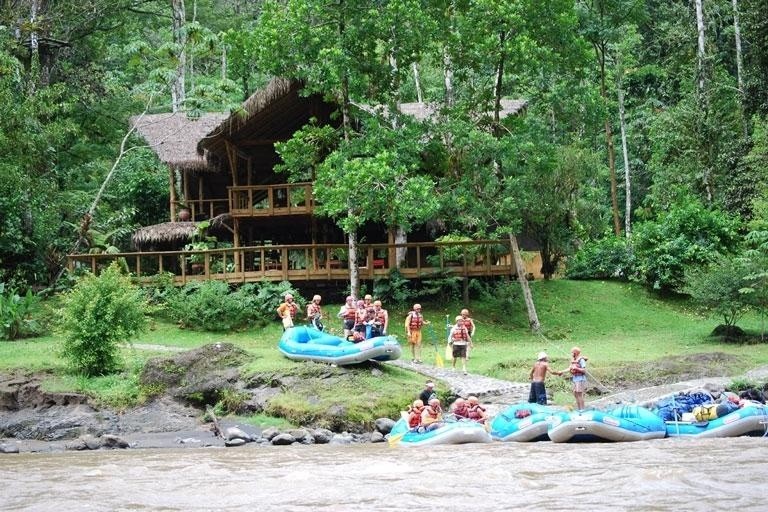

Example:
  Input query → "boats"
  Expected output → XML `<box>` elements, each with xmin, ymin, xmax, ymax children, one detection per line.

<box><xmin>491</xmin><ymin>400</ymin><xmax>572</xmax><ymax>444</ymax></box>
<box><xmin>281</xmin><ymin>325</ymin><xmax>403</xmax><ymax>368</ymax></box>
<box><xmin>390</xmin><ymin>414</ymin><xmax>493</xmax><ymax>446</ymax></box>
<box><xmin>649</xmin><ymin>398</ymin><xmax>768</xmax><ymax>438</ymax></box>
<box><xmin>548</xmin><ymin>401</ymin><xmax>667</xmax><ymax>447</ymax></box>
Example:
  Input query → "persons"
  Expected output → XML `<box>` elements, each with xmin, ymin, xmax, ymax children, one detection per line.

<box><xmin>449</xmin><ymin>309</ymin><xmax>476</xmax><ymax>375</ymax></box>
<box><xmin>407</xmin><ymin>379</ymin><xmax>488</xmax><ymax>435</ymax></box>
<box><xmin>306</xmin><ymin>294</ymin><xmax>324</xmax><ymax>330</ymax></box>
<box><xmin>276</xmin><ymin>294</ymin><xmax>298</xmax><ymax>332</ymax></box>
<box><xmin>404</xmin><ymin>304</ymin><xmax>431</xmax><ymax>363</ymax></box>
<box><xmin>528</xmin><ymin>351</ymin><xmax>562</xmax><ymax>405</ymax></box>
<box><xmin>561</xmin><ymin>348</ymin><xmax>587</xmax><ymax>411</ymax></box>
<box><xmin>336</xmin><ymin>294</ymin><xmax>389</xmax><ymax>344</ymax></box>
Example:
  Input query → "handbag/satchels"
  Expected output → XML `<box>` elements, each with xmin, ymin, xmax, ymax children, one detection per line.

<box><xmin>693</xmin><ymin>403</ymin><xmax>729</xmax><ymax>422</ymax></box>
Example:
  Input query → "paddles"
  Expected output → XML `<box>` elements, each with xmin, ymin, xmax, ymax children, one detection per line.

<box><xmin>445</xmin><ymin>314</ymin><xmax>453</xmax><ymax>360</ymax></box>
<box><xmin>388</xmin><ymin>431</ymin><xmax>410</xmax><ymax>447</ymax></box>
<box><xmin>666</xmin><ymin>421</ymin><xmax>708</xmax><ymax>427</ymax></box>
<box><xmin>427</xmin><ymin>319</ymin><xmax>444</xmax><ymax>366</ymax></box>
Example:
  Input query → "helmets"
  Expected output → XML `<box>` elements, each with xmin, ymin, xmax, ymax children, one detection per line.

<box><xmin>454</xmin><ymin>396</ymin><xmax>466</xmax><ymax>408</ymax></box>
<box><xmin>364</xmin><ymin>294</ymin><xmax>373</xmax><ymax>300</ymax></box>
<box><xmin>412</xmin><ymin>398</ymin><xmax>424</xmax><ymax>407</ymax></box>
<box><xmin>460</xmin><ymin>307</ymin><xmax>470</xmax><ymax>316</ymax></box>
<box><xmin>345</xmin><ymin>295</ymin><xmax>354</xmax><ymax>302</ymax></box>
<box><xmin>570</xmin><ymin>346</ymin><xmax>582</xmax><ymax>355</ymax></box>
<box><xmin>468</xmin><ymin>395</ymin><xmax>479</xmax><ymax>405</ymax></box>
<box><xmin>312</xmin><ymin>294</ymin><xmax>322</xmax><ymax>302</ymax></box>
<box><xmin>373</xmin><ymin>300</ymin><xmax>383</xmax><ymax>307</ymax></box>
<box><xmin>356</xmin><ymin>299</ymin><xmax>365</xmax><ymax>306</ymax></box>
<box><xmin>427</xmin><ymin>398</ymin><xmax>441</xmax><ymax>408</ymax></box>
<box><xmin>285</xmin><ymin>293</ymin><xmax>294</xmax><ymax>302</ymax></box>
<box><xmin>413</xmin><ymin>303</ymin><xmax>422</xmax><ymax>310</ymax></box>
<box><xmin>454</xmin><ymin>315</ymin><xmax>465</xmax><ymax>322</ymax></box>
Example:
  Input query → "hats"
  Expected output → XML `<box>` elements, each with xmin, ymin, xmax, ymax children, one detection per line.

<box><xmin>537</xmin><ymin>351</ymin><xmax>548</xmax><ymax>360</ymax></box>
<box><xmin>425</xmin><ymin>381</ymin><xmax>435</xmax><ymax>388</ymax></box>
